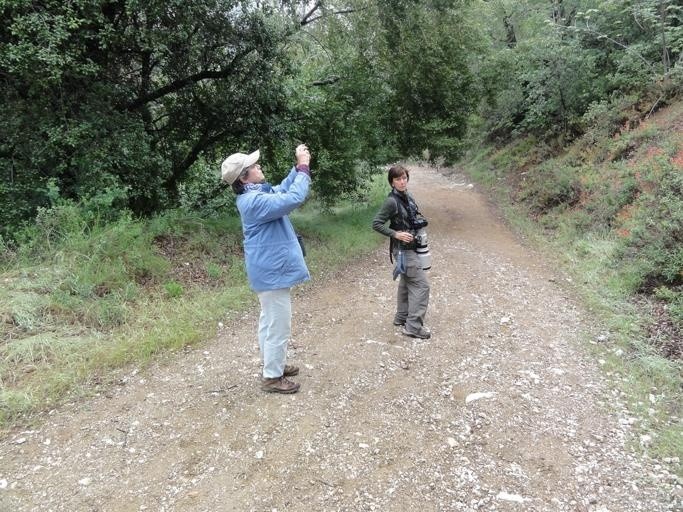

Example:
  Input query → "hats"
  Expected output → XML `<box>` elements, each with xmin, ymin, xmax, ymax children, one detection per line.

<box><xmin>221</xmin><ymin>149</ymin><xmax>260</xmax><ymax>185</ymax></box>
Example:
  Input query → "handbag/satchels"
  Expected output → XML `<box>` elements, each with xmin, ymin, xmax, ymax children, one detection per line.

<box><xmin>391</xmin><ymin>249</ymin><xmax>405</xmax><ymax>280</ymax></box>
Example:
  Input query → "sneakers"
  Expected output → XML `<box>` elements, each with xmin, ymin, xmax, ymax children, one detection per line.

<box><xmin>402</xmin><ymin>327</ymin><xmax>430</xmax><ymax>339</ymax></box>
<box><xmin>261</xmin><ymin>376</ymin><xmax>299</xmax><ymax>394</ymax></box>
<box><xmin>284</xmin><ymin>364</ymin><xmax>299</xmax><ymax>376</ymax></box>
<box><xmin>393</xmin><ymin>317</ymin><xmax>405</xmax><ymax>325</ymax></box>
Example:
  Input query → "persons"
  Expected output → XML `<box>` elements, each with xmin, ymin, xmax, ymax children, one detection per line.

<box><xmin>219</xmin><ymin>143</ymin><xmax>312</xmax><ymax>392</ymax></box>
<box><xmin>371</xmin><ymin>165</ymin><xmax>431</xmax><ymax>341</ymax></box>
